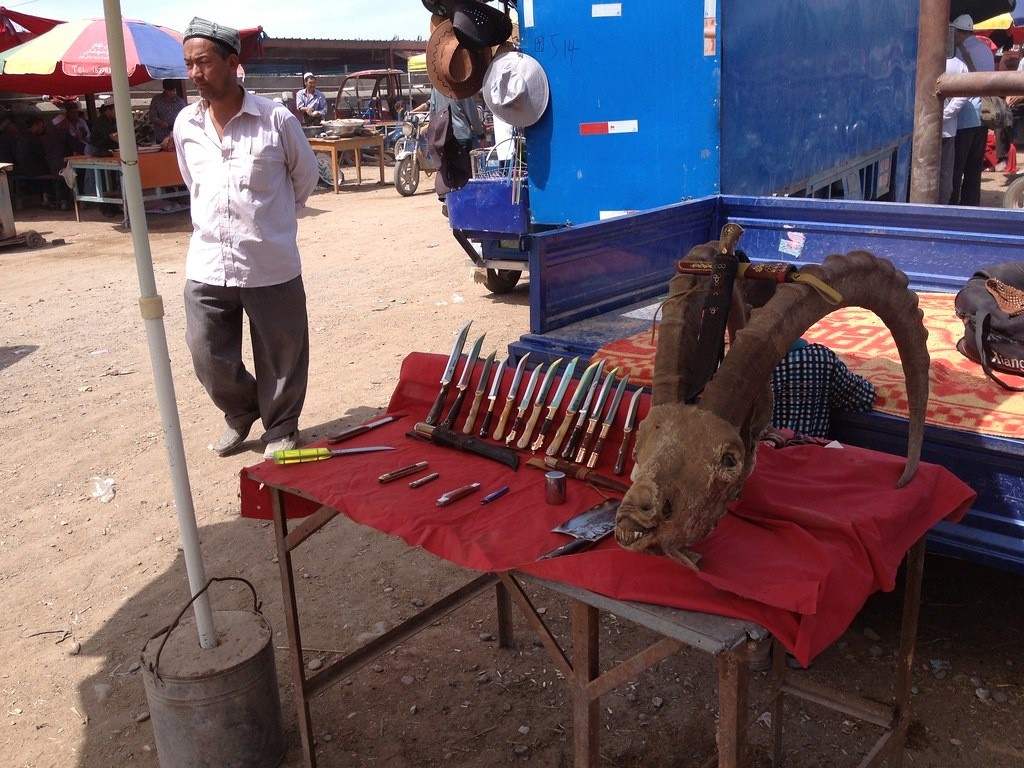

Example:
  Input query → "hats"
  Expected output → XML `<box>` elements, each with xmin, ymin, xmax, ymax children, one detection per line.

<box><xmin>103</xmin><ymin>96</ymin><xmax>114</xmax><ymax>107</ymax></box>
<box><xmin>447</xmin><ymin>0</ymin><xmax>513</xmax><ymax>51</ymax></box>
<box><xmin>303</xmin><ymin>71</ymin><xmax>314</xmax><ymax>79</ymax></box>
<box><xmin>425</xmin><ymin>18</ymin><xmax>493</xmax><ymax>100</ymax></box>
<box><xmin>948</xmin><ymin>14</ymin><xmax>974</xmax><ymax>32</ymax></box>
<box><xmin>420</xmin><ymin>0</ymin><xmax>491</xmax><ymax>18</ymax></box>
<box><xmin>425</xmin><ymin>102</ymin><xmax>454</xmax><ymax>170</ymax></box>
<box><xmin>479</xmin><ymin>23</ymin><xmax>522</xmax><ymax>68</ymax></box>
<box><xmin>182</xmin><ymin>16</ymin><xmax>241</xmax><ymax>58</ymax></box>
<box><xmin>482</xmin><ymin>50</ymin><xmax>549</xmax><ymax>129</ymax></box>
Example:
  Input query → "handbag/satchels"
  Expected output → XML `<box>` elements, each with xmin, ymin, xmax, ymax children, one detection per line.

<box><xmin>953</xmin><ymin>260</ymin><xmax>1024</xmax><ymax>393</ymax></box>
<box><xmin>979</xmin><ymin>92</ymin><xmax>1014</xmax><ymax>132</ymax></box>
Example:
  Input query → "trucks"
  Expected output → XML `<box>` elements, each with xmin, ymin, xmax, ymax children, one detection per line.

<box><xmin>443</xmin><ymin>0</ymin><xmax>921</xmax><ymax>298</ymax></box>
<box><xmin>503</xmin><ymin>195</ymin><xmax>1024</xmax><ymax>636</ymax></box>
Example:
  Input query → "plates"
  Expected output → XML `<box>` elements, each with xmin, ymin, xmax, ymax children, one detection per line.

<box><xmin>114</xmin><ymin>145</ymin><xmax>162</xmax><ymax>154</ymax></box>
<box><xmin>315</xmin><ymin>152</ymin><xmax>343</xmax><ymax>186</ymax></box>
<box><xmin>324</xmin><ymin>136</ymin><xmax>340</xmax><ymax>139</ymax></box>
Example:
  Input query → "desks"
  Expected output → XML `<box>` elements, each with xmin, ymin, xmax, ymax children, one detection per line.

<box><xmin>237</xmin><ymin>351</ymin><xmax>977</xmax><ymax>768</ymax></box>
<box><xmin>65</xmin><ymin>151</ymin><xmax>185</xmax><ymax>223</ymax></box>
<box><xmin>305</xmin><ymin>135</ymin><xmax>386</xmax><ymax>195</ymax></box>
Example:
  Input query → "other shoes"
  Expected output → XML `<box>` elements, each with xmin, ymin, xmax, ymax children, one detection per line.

<box><xmin>263</xmin><ymin>427</ymin><xmax>300</xmax><ymax>462</ymax></box>
<box><xmin>213</xmin><ymin>415</ymin><xmax>261</xmax><ymax>457</ymax></box>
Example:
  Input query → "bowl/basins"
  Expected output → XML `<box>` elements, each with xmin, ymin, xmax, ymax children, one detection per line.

<box><xmin>301</xmin><ymin>126</ymin><xmax>323</xmax><ymax>137</ymax></box>
<box><xmin>321</xmin><ymin>123</ymin><xmax>363</xmax><ymax>137</ymax></box>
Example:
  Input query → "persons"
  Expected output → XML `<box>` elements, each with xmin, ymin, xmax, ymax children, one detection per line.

<box><xmin>430</xmin><ymin>87</ymin><xmax>487</xmax><ymax>201</ymax></box>
<box><xmin>404</xmin><ymin>99</ymin><xmax>430</xmax><ymax>115</ymax></box>
<box><xmin>149</xmin><ymin>79</ymin><xmax>185</xmax><ymax>200</ymax></box>
<box><xmin>0</xmin><ymin>97</ymin><xmax>120</xmax><ymax>217</ymax></box>
<box><xmin>297</xmin><ymin>74</ymin><xmax>327</xmax><ymax>126</ymax></box>
<box><xmin>173</xmin><ymin>17</ymin><xmax>320</xmax><ymax>461</ymax></box>
<box><xmin>940</xmin><ymin>14</ymin><xmax>1024</xmax><ymax>207</ymax></box>
<box><xmin>395</xmin><ymin>101</ymin><xmax>404</xmax><ymax>132</ymax></box>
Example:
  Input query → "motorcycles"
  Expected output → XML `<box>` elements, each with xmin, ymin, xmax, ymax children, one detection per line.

<box><xmin>332</xmin><ymin>69</ymin><xmax>412</xmax><ymax>162</ymax></box>
<box><xmin>393</xmin><ymin>105</ymin><xmax>496</xmax><ymax>197</ymax></box>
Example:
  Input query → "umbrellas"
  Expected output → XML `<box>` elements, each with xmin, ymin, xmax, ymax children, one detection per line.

<box><xmin>974</xmin><ymin>12</ymin><xmax>1024</xmax><ymax>30</ymax></box>
<box><xmin>0</xmin><ymin>17</ymin><xmax>246</xmax><ymax>97</ymax></box>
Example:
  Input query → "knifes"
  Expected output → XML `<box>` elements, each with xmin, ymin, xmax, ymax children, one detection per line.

<box><xmin>506</xmin><ymin>362</ymin><xmax>544</xmax><ymax>447</ymax></box>
<box><xmin>463</xmin><ymin>349</ymin><xmax>498</xmax><ymax>432</ymax></box>
<box><xmin>440</xmin><ymin>332</ymin><xmax>490</xmax><ymax>426</ymax></box>
<box><xmin>275</xmin><ymin>445</ymin><xmax>397</xmax><ymax>464</ymax></box>
<box><xmin>546</xmin><ymin>361</ymin><xmax>600</xmax><ymax>456</ymax></box>
<box><xmin>328</xmin><ymin>415</ymin><xmax>410</xmax><ymax>444</ymax></box>
<box><xmin>613</xmin><ymin>387</ymin><xmax>643</xmax><ymax>475</ymax></box>
<box><xmin>517</xmin><ymin>357</ymin><xmax>564</xmax><ymax>450</ymax></box>
<box><xmin>492</xmin><ymin>351</ymin><xmax>531</xmax><ymax>440</ymax></box>
<box><xmin>544</xmin><ymin>359</ymin><xmax>632</xmax><ymax>493</ymax></box>
<box><xmin>479</xmin><ymin>354</ymin><xmax>510</xmax><ymax>435</ymax></box>
<box><xmin>535</xmin><ymin>497</ymin><xmax>626</xmax><ymax>562</ymax></box>
<box><xmin>425</xmin><ymin>320</ymin><xmax>473</xmax><ymax>424</ymax></box>
<box><xmin>415</xmin><ymin>421</ymin><xmax>523</xmax><ymax>472</ymax></box>
<box><xmin>379</xmin><ymin>461</ymin><xmax>509</xmax><ymax>507</ymax></box>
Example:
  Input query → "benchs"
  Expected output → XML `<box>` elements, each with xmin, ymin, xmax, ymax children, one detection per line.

<box><xmin>11</xmin><ymin>174</ymin><xmax>77</xmax><ymax>210</ymax></box>
